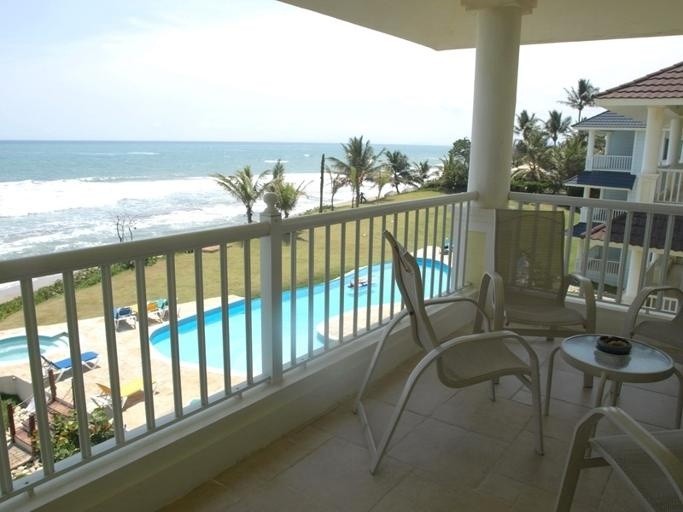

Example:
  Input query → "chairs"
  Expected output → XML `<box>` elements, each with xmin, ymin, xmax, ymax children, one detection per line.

<box><xmin>553</xmin><ymin>406</ymin><xmax>683</xmax><ymax>511</ymax></box>
<box><xmin>109</xmin><ymin>298</ymin><xmax>180</xmax><ymax>332</ymax></box>
<box><xmin>90</xmin><ymin>375</ymin><xmax>156</xmax><ymax>412</ymax></box>
<box><xmin>38</xmin><ymin>351</ymin><xmax>99</xmax><ymax>388</ymax></box>
<box><xmin>351</xmin><ymin>229</ymin><xmax>544</xmax><ymax>476</ymax></box>
<box><xmin>472</xmin><ymin>207</ymin><xmax>596</xmax><ymax>402</ymax></box>
<box><xmin>617</xmin><ymin>285</ymin><xmax>682</xmax><ymax>430</ymax></box>
